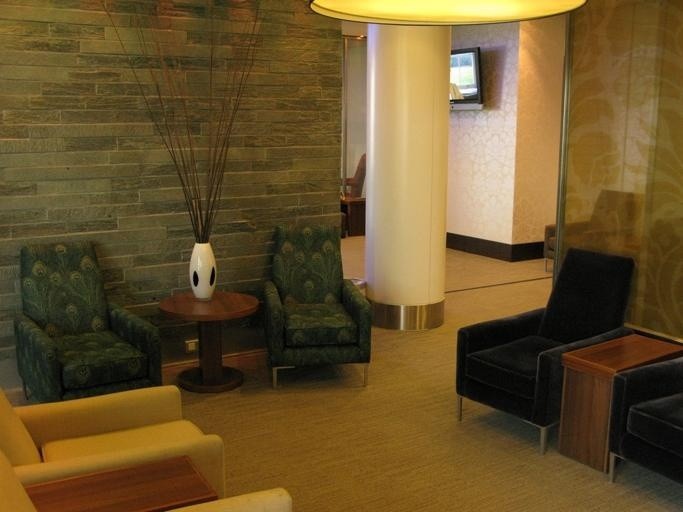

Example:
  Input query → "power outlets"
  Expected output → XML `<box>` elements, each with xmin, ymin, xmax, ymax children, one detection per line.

<box><xmin>184</xmin><ymin>339</ymin><xmax>199</xmax><ymax>354</ymax></box>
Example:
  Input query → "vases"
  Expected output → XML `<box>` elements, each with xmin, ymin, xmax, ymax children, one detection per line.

<box><xmin>189</xmin><ymin>242</ymin><xmax>217</xmax><ymax>300</ymax></box>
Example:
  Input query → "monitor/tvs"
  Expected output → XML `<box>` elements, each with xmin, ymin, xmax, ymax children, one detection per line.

<box><xmin>449</xmin><ymin>47</ymin><xmax>484</xmax><ymax>111</ymax></box>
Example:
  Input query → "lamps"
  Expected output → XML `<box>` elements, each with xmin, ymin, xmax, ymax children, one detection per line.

<box><xmin>303</xmin><ymin>0</ymin><xmax>591</xmax><ymax>29</ymax></box>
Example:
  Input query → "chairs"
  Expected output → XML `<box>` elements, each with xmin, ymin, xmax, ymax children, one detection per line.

<box><xmin>451</xmin><ymin>245</ymin><xmax>636</xmax><ymax>457</ymax></box>
<box><xmin>0</xmin><ymin>375</ymin><xmax>225</xmax><ymax>503</ymax></box>
<box><xmin>10</xmin><ymin>237</ymin><xmax>165</xmax><ymax>403</ymax></box>
<box><xmin>340</xmin><ymin>152</ymin><xmax>366</xmax><ymax>198</ymax></box>
<box><xmin>607</xmin><ymin>349</ymin><xmax>681</xmax><ymax>501</ymax></box>
<box><xmin>541</xmin><ymin>187</ymin><xmax>637</xmax><ymax>276</ymax></box>
<box><xmin>261</xmin><ymin>222</ymin><xmax>374</xmax><ymax>389</ymax></box>
<box><xmin>0</xmin><ymin>451</ymin><xmax>295</xmax><ymax>512</ymax></box>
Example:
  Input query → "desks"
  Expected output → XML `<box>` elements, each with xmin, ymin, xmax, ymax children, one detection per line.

<box><xmin>340</xmin><ymin>198</ymin><xmax>365</xmax><ymax>237</ymax></box>
<box><xmin>557</xmin><ymin>330</ymin><xmax>683</xmax><ymax>471</ymax></box>
<box><xmin>157</xmin><ymin>290</ymin><xmax>258</xmax><ymax>396</ymax></box>
